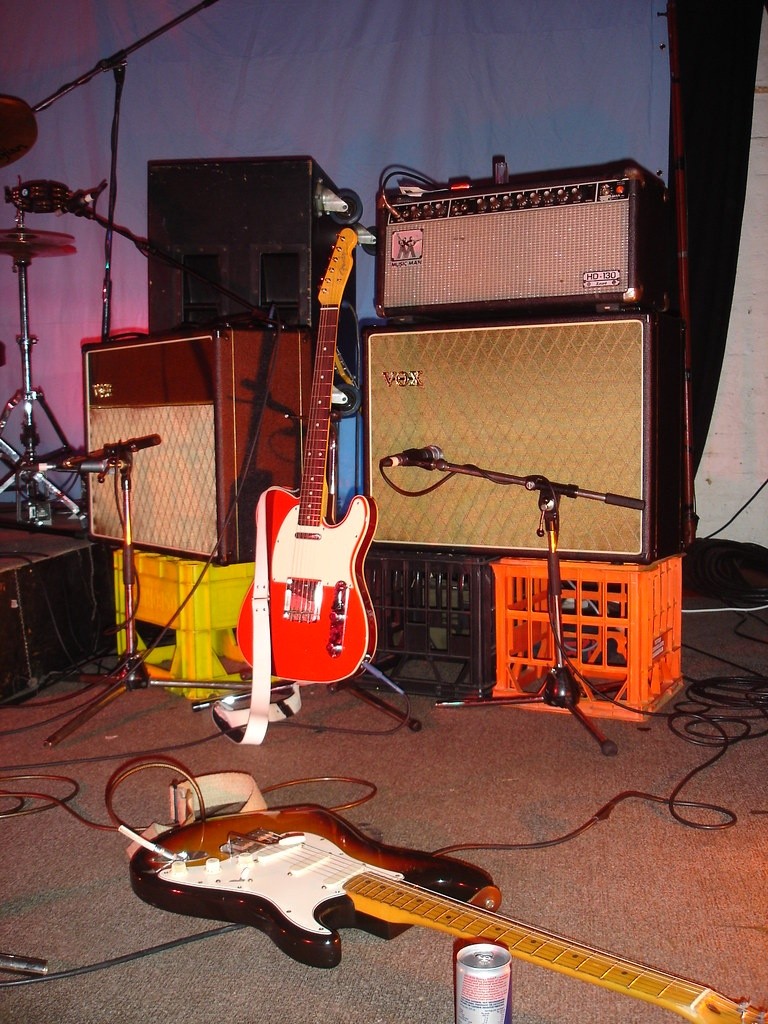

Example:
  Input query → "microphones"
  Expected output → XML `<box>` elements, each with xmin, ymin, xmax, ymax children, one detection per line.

<box><xmin>38</xmin><ymin>458</ymin><xmax>116</xmax><ymax>474</ymax></box>
<box><xmin>381</xmin><ymin>445</ymin><xmax>443</xmax><ymax>468</ymax></box>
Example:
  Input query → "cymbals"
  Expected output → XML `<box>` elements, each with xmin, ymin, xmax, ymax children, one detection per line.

<box><xmin>0</xmin><ymin>95</ymin><xmax>38</xmax><ymax>167</ymax></box>
<box><xmin>0</xmin><ymin>229</ymin><xmax>76</xmax><ymax>257</ymax></box>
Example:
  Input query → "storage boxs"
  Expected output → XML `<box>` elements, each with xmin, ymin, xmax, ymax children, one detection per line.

<box><xmin>364</xmin><ymin>548</ymin><xmax>495</xmax><ymax>700</ymax></box>
<box><xmin>110</xmin><ymin>551</ymin><xmax>256</xmax><ymax>702</ymax></box>
<box><xmin>495</xmin><ymin>557</ymin><xmax>682</xmax><ymax>724</ymax></box>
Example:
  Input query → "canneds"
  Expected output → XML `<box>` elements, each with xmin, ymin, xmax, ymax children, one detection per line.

<box><xmin>495</xmin><ymin>162</ymin><xmax>509</xmax><ymax>184</ymax></box>
<box><xmin>455</xmin><ymin>943</ymin><xmax>512</xmax><ymax>1024</ymax></box>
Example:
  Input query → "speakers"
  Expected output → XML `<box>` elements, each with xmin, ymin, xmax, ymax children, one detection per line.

<box><xmin>79</xmin><ymin>158</ymin><xmax>680</xmax><ymax>566</ymax></box>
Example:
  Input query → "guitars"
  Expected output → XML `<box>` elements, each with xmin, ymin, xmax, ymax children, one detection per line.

<box><xmin>130</xmin><ymin>802</ymin><xmax>768</xmax><ymax>1023</ymax></box>
<box><xmin>240</xmin><ymin>226</ymin><xmax>379</xmax><ymax>680</ymax></box>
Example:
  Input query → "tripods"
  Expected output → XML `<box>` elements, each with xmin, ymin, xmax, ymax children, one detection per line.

<box><xmin>0</xmin><ymin>252</ymin><xmax>86</xmax><ymax>491</ymax></box>
<box><xmin>43</xmin><ymin>433</ymin><xmax>295</xmax><ymax>750</ymax></box>
<box><xmin>191</xmin><ymin>420</ymin><xmax>424</xmax><ymax>733</ymax></box>
<box><xmin>414</xmin><ymin>457</ymin><xmax>645</xmax><ymax>756</ymax></box>
